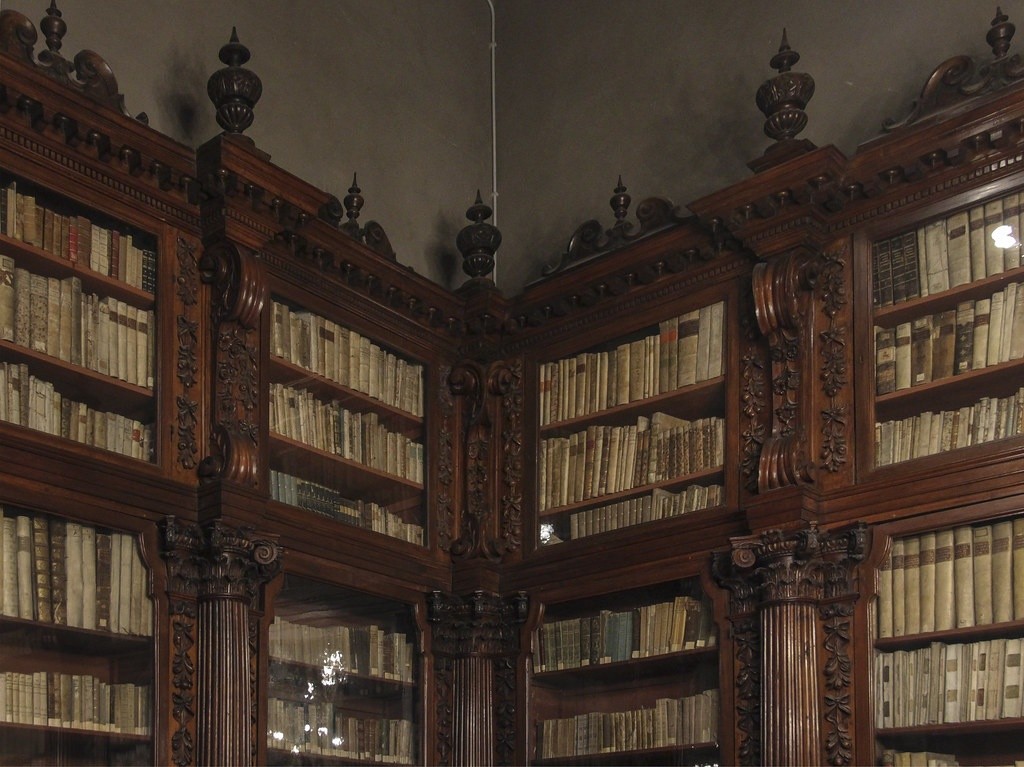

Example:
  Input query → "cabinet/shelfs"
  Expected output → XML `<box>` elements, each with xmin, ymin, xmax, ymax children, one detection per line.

<box><xmin>0</xmin><ymin>0</ymin><xmax>1024</xmax><ymax>767</ymax></box>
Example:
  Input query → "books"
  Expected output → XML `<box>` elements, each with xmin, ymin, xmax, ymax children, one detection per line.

<box><xmin>874</xmin><ymin>387</ymin><xmax>1024</xmax><ymax>470</ymax></box>
<box><xmin>0</xmin><ymin>361</ymin><xmax>151</xmax><ymax>462</ymax></box>
<box><xmin>535</xmin><ymin>688</ymin><xmax>718</xmax><ymax>760</ymax></box>
<box><xmin>0</xmin><ymin>254</ymin><xmax>156</xmax><ymax>392</ymax></box>
<box><xmin>269</xmin><ymin>379</ymin><xmax>423</xmax><ymax>486</ymax></box>
<box><xmin>539</xmin><ymin>303</ymin><xmax>724</xmax><ymax>425</ymax></box>
<box><xmin>873</xmin><ymin>280</ymin><xmax>1024</xmax><ymax>398</ymax></box>
<box><xmin>270</xmin><ymin>468</ymin><xmax>425</xmax><ymax>549</ymax></box>
<box><xmin>539</xmin><ymin>483</ymin><xmax>722</xmax><ymax>547</ymax></box>
<box><xmin>270</xmin><ymin>299</ymin><xmax>424</xmax><ymax>421</ymax></box>
<box><xmin>882</xmin><ymin>747</ymin><xmax>1024</xmax><ymax>767</ymax></box>
<box><xmin>532</xmin><ymin>596</ymin><xmax>717</xmax><ymax>675</ymax></box>
<box><xmin>872</xmin><ymin>189</ymin><xmax>1024</xmax><ymax>309</ymax></box>
<box><xmin>873</xmin><ymin>635</ymin><xmax>1023</xmax><ymax>731</ymax></box>
<box><xmin>537</xmin><ymin>411</ymin><xmax>725</xmax><ymax>511</ymax></box>
<box><xmin>0</xmin><ymin>506</ymin><xmax>155</xmax><ymax>739</ymax></box>
<box><xmin>870</xmin><ymin>515</ymin><xmax>1024</xmax><ymax>641</ymax></box>
<box><xmin>0</xmin><ymin>180</ymin><xmax>155</xmax><ymax>296</ymax></box>
<box><xmin>267</xmin><ymin>615</ymin><xmax>416</xmax><ymax>765</ymax></box>
